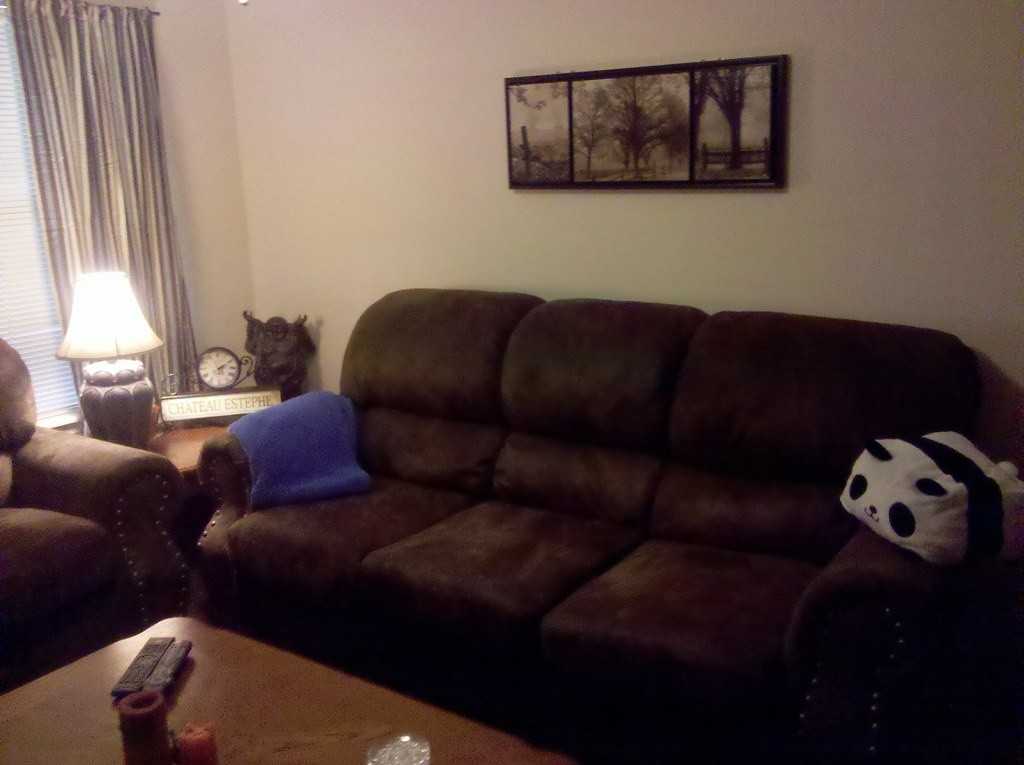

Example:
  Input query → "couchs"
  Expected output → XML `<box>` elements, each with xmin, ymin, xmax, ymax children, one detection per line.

<box><xmin>1</xmin><ymin>339</ymin><xmax>192</xmax><ymax>692</ymax></box>
<box><xmin>192</xmin><ymin>288</ymin><xmax>1023</xmax><ymax>765</ymax></box>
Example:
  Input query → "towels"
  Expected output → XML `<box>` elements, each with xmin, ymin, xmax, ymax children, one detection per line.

<box><xmin>226</xmin><ymin>389</ymin><xmax>373</xmax><ymax>513</ymax></box>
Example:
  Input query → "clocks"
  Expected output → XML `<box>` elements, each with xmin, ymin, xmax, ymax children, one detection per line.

<box><xmin>194</xmin><ymin>346</ymin><xmax>241</xmax><ymax>391</ymax></box>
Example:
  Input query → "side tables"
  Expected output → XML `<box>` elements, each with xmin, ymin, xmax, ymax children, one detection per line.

<box><xmin>146</xmin><ymin>424</ymin><xmax>228</xmax><ymax>490</ymax></box>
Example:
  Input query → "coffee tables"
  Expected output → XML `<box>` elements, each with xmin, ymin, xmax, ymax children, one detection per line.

<box><xmin>1</xmin><ymin>617</ymin><xmax>580</xmax><ymax>765</ymax></box>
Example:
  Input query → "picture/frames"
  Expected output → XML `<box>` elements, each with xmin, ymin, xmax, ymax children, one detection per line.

<box><xmin>503</xmin><ymin>53</ymin><xmax>790</xmax><ymax>191</ymax></box>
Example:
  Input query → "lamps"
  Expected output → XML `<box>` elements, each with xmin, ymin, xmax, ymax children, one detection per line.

<box><xmin>55</xmin><ymin>270</ymin><xmax>164</xmax><ymax>451</ymax></box>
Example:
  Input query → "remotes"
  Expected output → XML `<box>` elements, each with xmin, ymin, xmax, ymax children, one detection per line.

<box><xmin>110</xmin><ymin>636</ymin><xmax>193</xmax><ymax>698</ymax></box>
